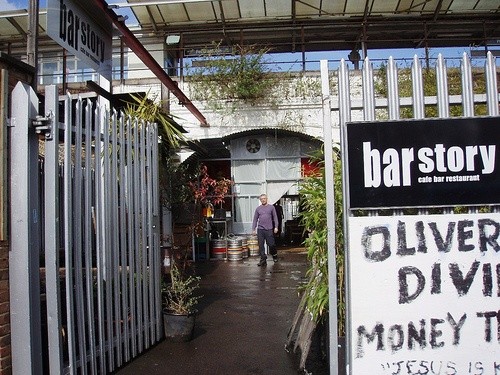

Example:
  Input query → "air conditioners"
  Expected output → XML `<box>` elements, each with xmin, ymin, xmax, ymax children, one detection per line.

<box><xmin>230</xmin><ymin>135</ymin><xmax>266</xmax><ymax>161</ymax></box>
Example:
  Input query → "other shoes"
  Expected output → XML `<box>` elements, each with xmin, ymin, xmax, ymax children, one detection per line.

<box><xmin>257</xmin><ymin>259</ymin><xmax>266</xmax><ymax>266</ymax></box>
<box><xmin>272</xmin><ymin>253</ymin><xmax>278</xmax><ymax>262</ymax></box>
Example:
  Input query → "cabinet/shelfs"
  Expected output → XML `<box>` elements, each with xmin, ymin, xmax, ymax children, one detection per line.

<box><xmin>206</xmin><ymin>218</ymin><xmax>233</xmax><ymax>261</ymax></box>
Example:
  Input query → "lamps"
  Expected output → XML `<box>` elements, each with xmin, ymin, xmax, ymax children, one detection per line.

<box><xmin>165</xmin><ymin>32</ymin><xmax>181</xmax><ymax>45</ymax></box>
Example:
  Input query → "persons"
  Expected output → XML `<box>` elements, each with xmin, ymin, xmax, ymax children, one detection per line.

<box><xmin>252</xmin><ymin>194</ymin><xmax>278</xmax><ymax>266</ymax></box>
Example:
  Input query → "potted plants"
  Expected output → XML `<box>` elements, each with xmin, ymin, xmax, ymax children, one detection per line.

<box><xmin>161</xmin><ymin>264</ymin><xmax>205</xmax><ymax>340</ymax></box>
